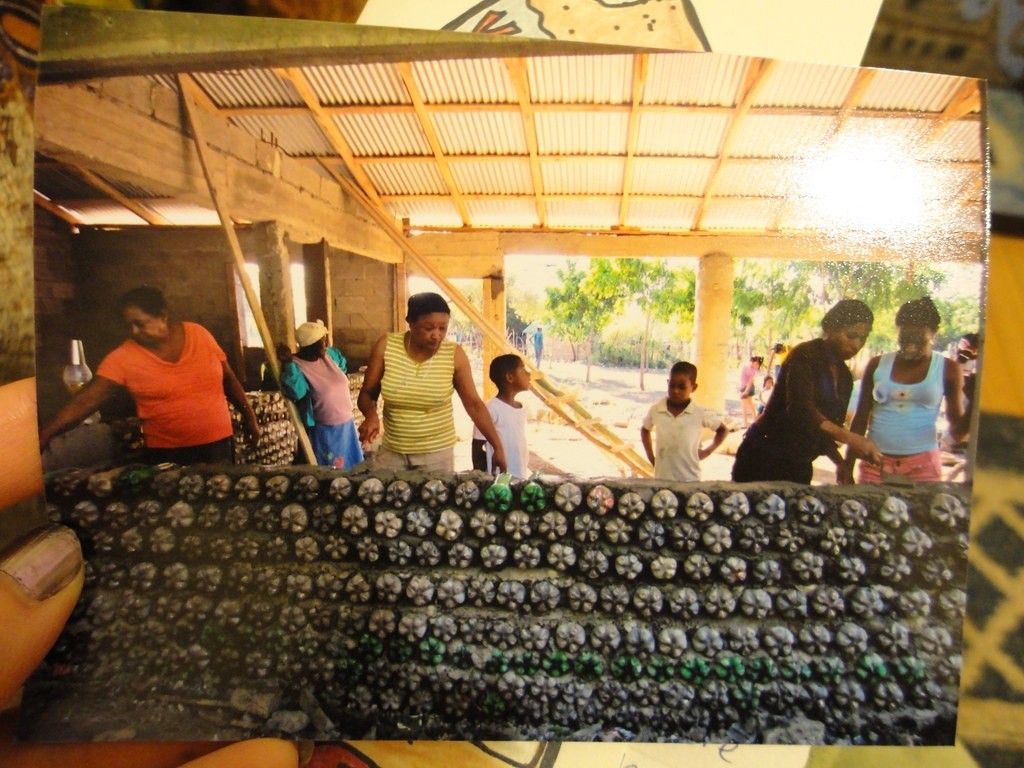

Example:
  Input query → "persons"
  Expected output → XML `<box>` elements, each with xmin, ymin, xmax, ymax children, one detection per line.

<box><xmin>280</xmin><ymin>322</ymin><xmax>364</xmax><ymax>469</ymax></box>
<box><xmin>358</xmin><ymin>292</ymin><xmax>508</xmax><ymax>476</ymax></box>
<box><xmin>732</xmin><ymin>300</ymin><xmax>884</xmax><ymax>483</ymax></box>
<box><xmin>739</xmin><ymin>343</ymin><xmax>793</xmax><ymax>426</ymax></box>
<box><xmin>942</xmin><ymin>332</ymin><xmax>980</xmax><ymax>454</ymax></box>
<box><xmin>529</xmin><ymin>327</ymin><xmax>543</xmax><ymax>369</ymax></box>
<box><xmin>843</xmin><ymin>298</ymin><xmax>976</xmax><ymax>485</ymax></box>
<box><xmin>39</xmin><ymin>286</ymin><xmax>260</xmax><ymax>464</ymax></box>
<box><xmin>641</xmin><ymin>361</ymin><xmax>729</xmax><ymax>480</ymax></box>
<box><xmin>472</xmin><ymin>355</ymin><xmax>531</xmax><ymax>480</ymax></box>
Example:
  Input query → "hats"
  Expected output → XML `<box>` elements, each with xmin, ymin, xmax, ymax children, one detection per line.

<box><xmin>295</xmin><ymin>319</ymin><xmax>327</xmax><ymax>347</ymax></box>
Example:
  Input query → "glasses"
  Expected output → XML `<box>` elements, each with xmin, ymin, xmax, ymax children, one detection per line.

<box><xmin>668</xmin><ymin>382</ymin><xmax>692</xmax><ymax>390</ymax></box>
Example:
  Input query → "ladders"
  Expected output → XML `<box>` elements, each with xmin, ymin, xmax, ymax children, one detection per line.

<box><xmin>316</xmin><ymin>156</ymin><xmax>659</xmax><ymax>478</ymax></box>
